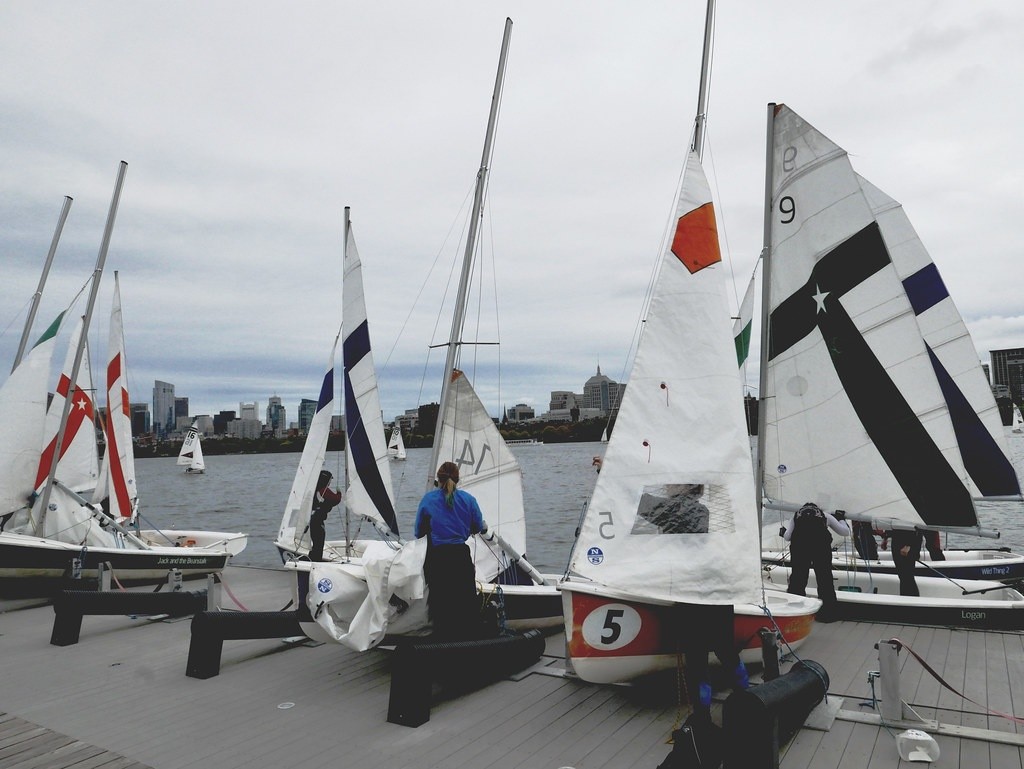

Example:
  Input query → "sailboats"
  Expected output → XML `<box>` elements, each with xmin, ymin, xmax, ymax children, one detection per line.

<box><xmin>1</xmin><ymin>159</ymin><xmax>250</xmax><ymax>616</ymax></box>
<box><xmin>387</xmin><ymin>421</ymin><xmax>407</xmax><ymax>461</ymax></box>
<box><xmin>1011</xmin><ymin>403</ymin><xmax>1024</xmax><ymax>433</ymax></box>
<box><xmin>178</xmin><ymin>420</ymin><xmax>206</xmax><ymax>474</ymax></box>
<box><xmin>557</xmin><ymin>2</ymin><xmax>826</xmax><ymax>683</ymax></box>
<box><xmin>733</xmin><ymin>97</ymin><xmax>1024</xmax><ymax>634</ymax></box>
<box><xmin>276</xmin><ymin>20</ymin><xmax>567</xmax><ymax>655</ymax></box>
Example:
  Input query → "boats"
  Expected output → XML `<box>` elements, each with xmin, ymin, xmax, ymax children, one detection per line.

<box><xmin>506</xmin><ymin>439</ymin><xmax>544</xmax><ymax>445</ymax></box>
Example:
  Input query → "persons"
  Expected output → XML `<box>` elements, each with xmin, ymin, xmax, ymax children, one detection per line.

<box><xmin>851</xmin><ymin>520</ymin><xmax>879</xmax><ymax>560</ymax></box>
<box><xmin>778</xmin><ymin>503</ymin><xmax>850</xmax><ymax>624</ymax></box>
<box><xmin>414</xmin><ymin>461</ymin><xmax>485</xmax><ymax>642</ymax></box>
<box><xmin>922</xmin><ymin>529</ymin><xmax>946</xmax><ymax>561</ymax></box>
<box><xmin>593</xmin><ymin>456</ymin><xmax>750</xmax><ymax>741</ymax></box>
<box><xmin>308</xmin><ymin>469</ymin><xmax>341</xmax><ymax>562</ymax></box>
<box><xmin>882</xmin><ymin>525</ymin><xmax>923</xmax><ymax>597</ymax></box>
